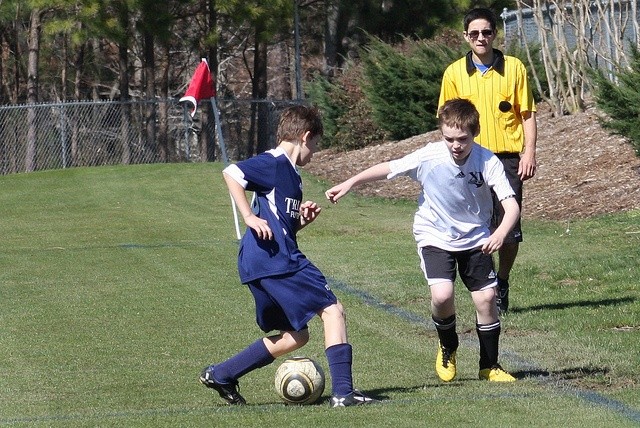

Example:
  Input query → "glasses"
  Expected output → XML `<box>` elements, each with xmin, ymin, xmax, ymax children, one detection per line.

<box><xmin>466</xmin><ymin>30</ymin><xmax>494</xmax><ymax>37</ymax></box>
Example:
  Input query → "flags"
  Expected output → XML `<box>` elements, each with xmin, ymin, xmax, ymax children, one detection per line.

<box><xmin>178</xmin><ymin>58</ymin><xmax>216</xmax><ymax>117</ymax></box>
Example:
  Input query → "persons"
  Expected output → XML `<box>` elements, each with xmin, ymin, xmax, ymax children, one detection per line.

<box><xmin>199</xmin><ymin>104</ymin><xmax>383</xmax><ymax>407</ymax></box>
<box><xmin>324</xmin><ymin>97</ymin><xmax>520</xmax><ymax>382</ymax></box>
<box><xmin>436</xmin><ymin>8</ymin><xmax>538</xmax><ymax>316</ymax></box>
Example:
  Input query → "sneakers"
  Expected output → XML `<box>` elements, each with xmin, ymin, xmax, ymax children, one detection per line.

<box><xmin>495</xmin><ymin>283</ymin><xmax>509</xmax><ymax>314</ymax></box>
<box><xmin>198</xmin><ymin>365</ymin><xmax>246</xmax><ymax>403</ymax></box>
<box><xmin>435</xmin><ymin>341</ymin><xmax>457</xmax><ymax>381</ymax></box>
<box><xmin>328</xmin><ymin>388</ymin><xmax>381</xmax><ymax>408</ymax></box>
<box><xmin>478</xmin><ymin>362</ymin><xmax>516</xmax><ymax>384</ymax></box>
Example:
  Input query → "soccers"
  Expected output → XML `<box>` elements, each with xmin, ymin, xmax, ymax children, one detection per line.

<box><xmin>274</xmin><ymin>356</ymin><xmax>325</xmax><ymax>406</ymax></box>
